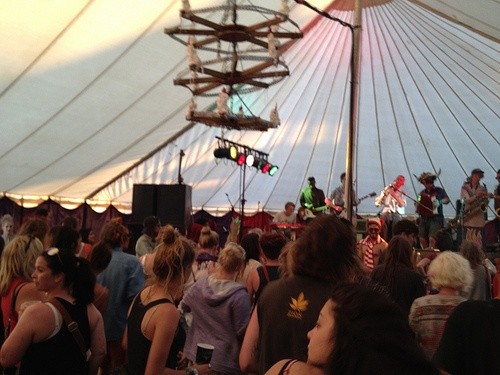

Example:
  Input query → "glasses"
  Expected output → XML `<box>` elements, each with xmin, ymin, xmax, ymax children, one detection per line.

<box><xmin>368</xmin><ymin>228</ymin><xmax>379</xmax><ymax>232</ymax></box>
<box><xmin>44</xmin><ymin>247</ymin><xmax>64</xmax><ymax>267</ymax></box>
<box><xmin>24</xmin><ymin>235</ymin><xmax>35</xmax><ymax>254</ymax></box>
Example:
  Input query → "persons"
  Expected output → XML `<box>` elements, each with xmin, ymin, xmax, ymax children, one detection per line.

<box><xmin>0</xmin><ymin>169</ymin><xmax>500</xmax><ymax>375</ymax></box>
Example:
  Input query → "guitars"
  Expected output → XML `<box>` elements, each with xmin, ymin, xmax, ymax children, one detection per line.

<box><xmin>330</xmin><ymin>192</ymin><xmax>376</xmax><ymax>217</ymax></box>
<box><xmin>298</xmin><ymin>204</ymin><xmax>329</xmax><ymax>222</ymax></box>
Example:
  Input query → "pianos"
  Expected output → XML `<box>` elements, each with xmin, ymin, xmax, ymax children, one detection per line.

<box><xmin>269</xmin><ymin>222</ymin><xmax>300</xmax><ymax>231</ymax></box>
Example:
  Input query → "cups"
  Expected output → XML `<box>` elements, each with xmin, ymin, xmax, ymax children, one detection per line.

<box><xmin>196</xmin><ymin>343</ymin><xmax>214</xmax><ymax>365</ymax></box>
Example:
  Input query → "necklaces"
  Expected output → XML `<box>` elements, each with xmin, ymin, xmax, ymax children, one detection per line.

<box><xmin>156</xmin><ymin>282</ymin><xmax>174</xmax><ymax>302</ymax></box>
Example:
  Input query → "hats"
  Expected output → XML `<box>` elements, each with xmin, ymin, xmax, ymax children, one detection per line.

<box><xmin>471</xmin><ymin>168</ymin><xmax>484</xmax><ymax>173</ymax></box>
<box><xmin>368</xmin><ymin>217</ymin><xmax>384</xmax><ymax>231</ymax></box>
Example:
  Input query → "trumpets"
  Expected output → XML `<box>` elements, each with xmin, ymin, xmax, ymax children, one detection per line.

<box><xmin>465</xmin><ymin>185</ymin><xmax>488</xmax><ymax>213</ymax></box>
